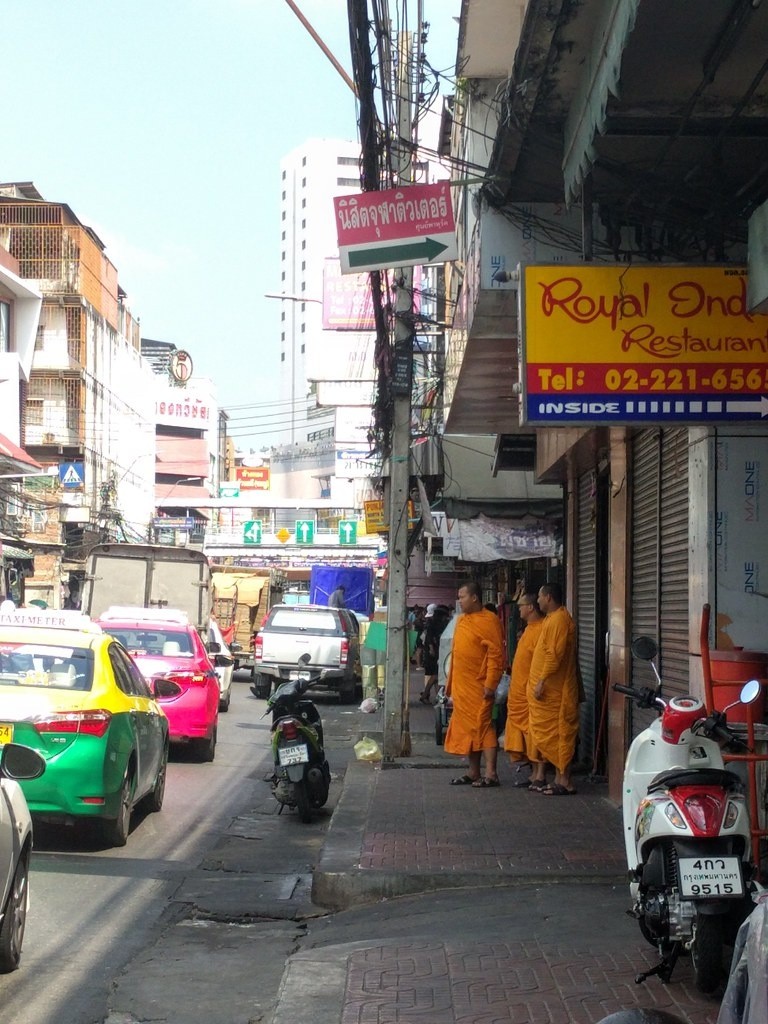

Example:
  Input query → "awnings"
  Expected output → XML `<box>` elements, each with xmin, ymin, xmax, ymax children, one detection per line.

<box><xmin>0</xmin><ymin>433</ymin><xmax>43</xmax><ymax>473</ymax></box>
<box><xmin>155</xmin><ymin>435</ymin><xmax>209</xmax><ymax>478</ymax></box>
<box><xmin>154</xmin><ymin>484</ymin><xmax>209</xmax><ymax>499</ymax></box>
<box><xmin>155</xmin><ymin>507</ymin><xmax>210</xmax><ymax>520</ymax></box>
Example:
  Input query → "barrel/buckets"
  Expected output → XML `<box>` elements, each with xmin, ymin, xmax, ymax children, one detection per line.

<box><xmin>708</xmin><ymin>647</ymin><xmax>768</xmax><ymax>723</ymax></box>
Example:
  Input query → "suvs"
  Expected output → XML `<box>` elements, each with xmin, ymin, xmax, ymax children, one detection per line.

<box><xmin>253</xmin><ymin>603</ymin><xmax>362</xmax><ymax>705</ymax></box>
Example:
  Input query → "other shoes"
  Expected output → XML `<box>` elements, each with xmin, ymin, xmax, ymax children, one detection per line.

<box><xmin>449</xmin><ymin>774</ymin><xmax>481</xmax><ymax>785</ymax></box>
<box><xmin>527</xmin><ymin>779</ymin><xmax>546</xmax><ymax>791</ymax></box>
<box><xmin>420</xmin><ymin>698</ymin><xmax>432</xmax><ymax>704</ymax></box>
<box><xmin>471</xmin><ymin>776</ymin><xmax>500</xmax><ymax>787</ymax></box>
<box><xmin>515</xmin><ymin>778</ymin><xmax>532</xmax><ymax>788</ymax></box>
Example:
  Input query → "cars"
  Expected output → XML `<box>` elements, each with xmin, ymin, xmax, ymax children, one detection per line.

<box><xmin>91</xmin><ymin>608</ymin><xmax>234</xmax><ymax>762</ymax></box>
<box><xmin>206</xmin><ymin>619</ymin><xmax>242</xmax><ymax>713</ymax></box>
<box><xmin>0</xmin><ymin>609</ymin><xmax>183</xmax><ymax>849</ymax></box>
<box><xmin>0</xmin><ymin>743</ymin><xmax>47</xmax><ymax>972</ymax></box>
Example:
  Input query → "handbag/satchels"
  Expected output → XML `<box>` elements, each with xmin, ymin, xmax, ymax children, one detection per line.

<box><xmin>354</xmin><ymin>736</ymin><xmax>382</xmax><ymax>762</ymax></box>
<box><xmin>494</xmin><ymin>674</ymin><xmax>510</xmax><ymax>704</ymax></box>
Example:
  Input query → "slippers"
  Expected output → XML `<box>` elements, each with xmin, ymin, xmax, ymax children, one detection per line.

<box><xmin>537</xmin><ymin>783</ymin><xmax>559</xmax><ymax>792</ymax></box>
<box><xmin>543</xmin><ymin>783</ymin><xmax>576</xmax><ymax>795</ymax></box>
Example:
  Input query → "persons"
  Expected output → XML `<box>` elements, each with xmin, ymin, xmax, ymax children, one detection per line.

<box><xmin>443</xmin><ymin>582</ymin><xmax>507</xmax><ymax>788</ymax></box>
<box><xmin>527</xmin><ymin>583</ymin><xmax>586</xmax><ymax>795</ymax></box>
<box><xmin>329</xmin><ymin>586</ymin><xmax>345</xmax><ymax>608</ymax></box>
<box><xmin>409</xmin><ymin>604</ymin><xmax>498</xmax><ymax>705</ymax></box>
<box><xmin>504</xmin><ymin>591</ymin><xmax>547</xmax><ymax>790</ymax></box>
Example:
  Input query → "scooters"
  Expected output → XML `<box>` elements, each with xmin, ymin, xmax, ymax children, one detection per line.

<box><xmin>263</xmin><ymin>654</ymin><xmax>332</xmax><ymax>822</ymax></box>
<box><xmin>612</xmin><ymin>636</ymin><xmax>762</xmax><ymax>985</ymax></box>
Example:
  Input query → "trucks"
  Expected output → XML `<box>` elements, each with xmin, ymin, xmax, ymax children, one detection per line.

<box><xmin>80</xmin><ymin>542</ymin><xmax>221</xmax><ymax>654</ymax></box>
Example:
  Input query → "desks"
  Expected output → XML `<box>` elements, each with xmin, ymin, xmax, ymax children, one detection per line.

<box><xmin>726</xmin><ymin>720</ymin><xmax>768</xmax><ymax>862</ymax></box>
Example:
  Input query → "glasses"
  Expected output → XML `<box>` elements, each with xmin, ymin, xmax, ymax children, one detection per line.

<box><xmin>518</xmin><ymin>604</ymin><xmax>530</xmax><ymax>607</ymax></box>
